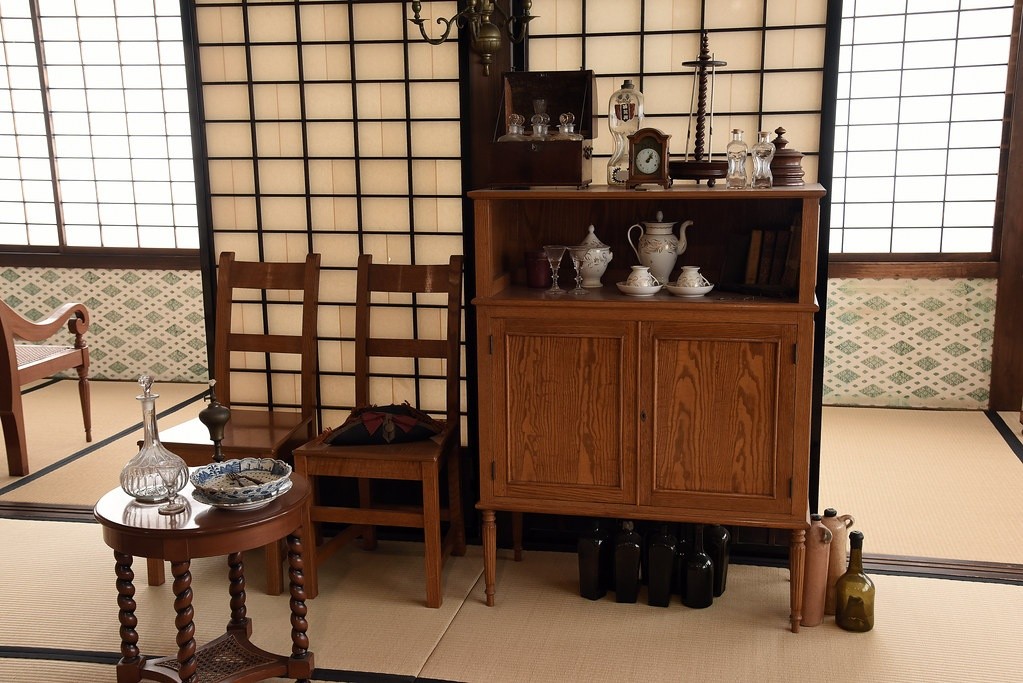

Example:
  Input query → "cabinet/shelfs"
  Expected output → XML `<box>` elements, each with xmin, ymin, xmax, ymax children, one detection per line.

<box><xmin>467</xmin><ymin>184</ymin><xmax>827</xmax><ymax>633</ymax></box>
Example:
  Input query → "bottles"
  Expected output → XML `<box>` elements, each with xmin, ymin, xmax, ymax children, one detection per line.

<box><xmin>833</xmin><ymin>531</ymin><xmax>875</xmax><ymax>632</ymax></box>
<box><xmin>799</xmin><ymin>508</ymin><xmax>855</xmax><ymax>627</ymax></box>
<box><xmin>726</xmin><ymin>129</ymin><xmax>749</xmax><ymax>190</ymax></box>
<box><xmin>496</xmin><ymin>112</ymin><xmax>584</xmax><ymax>141</ymax></box>
<box><xmin>750</xmin><ymin>132</ymin><xmax>776</xmax><ymax>190</ymax></box>
<box><xmin>578</xmin><ymin>516</ymin><xmax>730</xmax><ymax>609</ymax></box>
<box><xmin>576</xmin><ymin>225</ymin><xmax>613</xmax><ymax>288</ymax></box>
<box><xmin>607</xmin><ymin>79</ymin><xmax>644</xmax><ymax>187</ymax></box>
<box><xmin>120</xmin><ymin>374</ymin><xmax>189</xmax><ymax>506</ymax></box>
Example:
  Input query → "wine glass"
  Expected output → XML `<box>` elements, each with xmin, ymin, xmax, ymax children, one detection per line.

<box><xmin>567</xmin><ymin>245</ymin><xmax>592</xmax><ymax>295</ymax></box>
<box><xmin>154</xmin><ymin>459</ymin><xmax>186</xmax><ymax>516</ymax></box>
<box><xmin>543</xmin><ymin>244</ymin><xmax>568</xmax><ymax>295</ymax></box>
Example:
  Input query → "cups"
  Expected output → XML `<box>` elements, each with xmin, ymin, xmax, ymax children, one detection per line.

<box><xmin>626</xmin><ymin>265</ymin><xmax>660</xmax><ymax>287</ymax></box>
<box><xmin>676</xmin><ymin>266</ymin><xmax>710</xmax><ymax>288</ymax></box>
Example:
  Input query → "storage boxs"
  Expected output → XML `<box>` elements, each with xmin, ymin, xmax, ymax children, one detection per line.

<box><xmin>490</xmin><ymin>70</ymin><xmax>599</xmax><ymax>190</ymax></box>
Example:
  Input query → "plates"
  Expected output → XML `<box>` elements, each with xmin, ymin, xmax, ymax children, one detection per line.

<box><xmin>665</xmin><ymin>283</ymin><xmax>714</xmax><ymax>297</ymax></box>
<box><xmin>189</xmin><ymin>457</ymin><xmax>292</xmax><ymax>501</ymax></box>
<box><xmin>191</xmin><ymin>478</ymin><xmax>294</xmax><ymax>511</ymax></box>
<box><xmin>616</xmin><ymin>281</ymin><xmax>663</xmax><ymax>296</ymax></box>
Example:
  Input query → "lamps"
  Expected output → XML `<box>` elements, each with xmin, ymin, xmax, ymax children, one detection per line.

<box><xmin>406</xmin><ymin>0</ymin><xmax>541</xmax><ymax>75</ymax></box>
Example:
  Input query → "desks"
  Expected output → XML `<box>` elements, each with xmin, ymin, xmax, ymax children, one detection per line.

<box><xmin>93</xmin><ymin>467</ymin><xmax>314</xmax><ymax>683</ymax></box>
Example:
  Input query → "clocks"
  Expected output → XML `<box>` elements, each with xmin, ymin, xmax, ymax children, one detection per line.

<box><xmin>626</xmin><ymin>128</ymin><xmax>672</xmax><ymax>189</ymax></box>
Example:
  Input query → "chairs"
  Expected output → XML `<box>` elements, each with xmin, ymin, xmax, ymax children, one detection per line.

<box><xmin>137</xmin><ymin>252</ymin><xmax>321</xmax><ymax>596</ymax></box>
<box><xmin>292</xmin><ymin>254</ymin><xmax>464</xmax><ymax>608</ymax></box>
<box><xmin>0</xmin><ymin>298</ymin><xmax>92</xmax><ymax>476</ymax></box>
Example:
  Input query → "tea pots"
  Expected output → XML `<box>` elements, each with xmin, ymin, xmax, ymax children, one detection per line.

<box><xmin>627</xmin><ymin>210</ymin><xmax>694</xmax><ymax>289</ymax></box>
